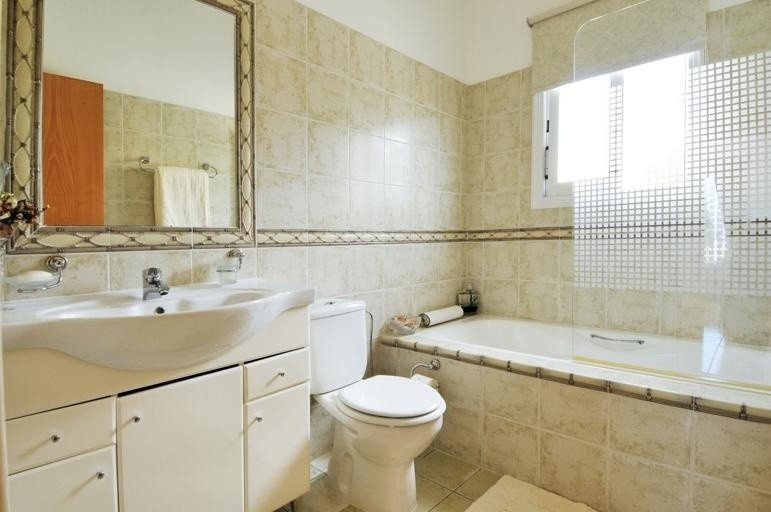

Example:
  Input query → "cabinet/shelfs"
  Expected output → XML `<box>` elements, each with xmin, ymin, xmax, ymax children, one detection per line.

<box><xmin>2</xmin><ymin>307</ymin><xmax>313</xmax><ymax>512</ymax></box>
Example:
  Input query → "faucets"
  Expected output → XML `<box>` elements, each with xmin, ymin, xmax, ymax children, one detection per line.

<box><xmin>143</xmin><ymin>268</ymin><xmax>170</xmax><ymax>300</ymax></box>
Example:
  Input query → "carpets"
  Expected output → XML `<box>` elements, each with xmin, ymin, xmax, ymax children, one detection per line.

<box><xmin>464</xmin><ymin>469</ymin><xmax>599</xmax><ymax>510</ymax></box>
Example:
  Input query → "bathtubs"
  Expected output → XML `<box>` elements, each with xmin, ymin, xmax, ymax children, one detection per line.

<box><xmin>379</xmin><ymin>314</ymin><xmax>771</xmax><ymax>424</ymax></box>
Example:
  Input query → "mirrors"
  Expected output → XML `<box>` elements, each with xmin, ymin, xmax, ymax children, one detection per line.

<box><xmin>5</xmin><ymin>0</ymin><xmax>256</xmax><ymax>255</ymax></box>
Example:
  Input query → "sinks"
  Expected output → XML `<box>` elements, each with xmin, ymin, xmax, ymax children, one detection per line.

<box><xmin>37</xmin><ymin>290</ymin><xmax>269</xmax><ymax>374</ymax></box>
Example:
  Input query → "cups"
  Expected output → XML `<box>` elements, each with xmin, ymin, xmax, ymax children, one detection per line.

<box><xmin>216</xmin><ymin>265</ymin><xmax>238</xmax><ymax>285</ymax></box>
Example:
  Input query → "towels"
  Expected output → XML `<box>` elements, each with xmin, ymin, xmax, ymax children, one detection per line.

<box><xmin>150</xmin><ymin>162</ymin><xmax>214</xmax><ymax>230</ymax></box>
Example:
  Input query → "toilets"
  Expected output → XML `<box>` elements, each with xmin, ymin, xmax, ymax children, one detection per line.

<box><xmin>309</xmin><ymin>298</ymin><xmax>446</xmax><ymax>512</ymax></box>
